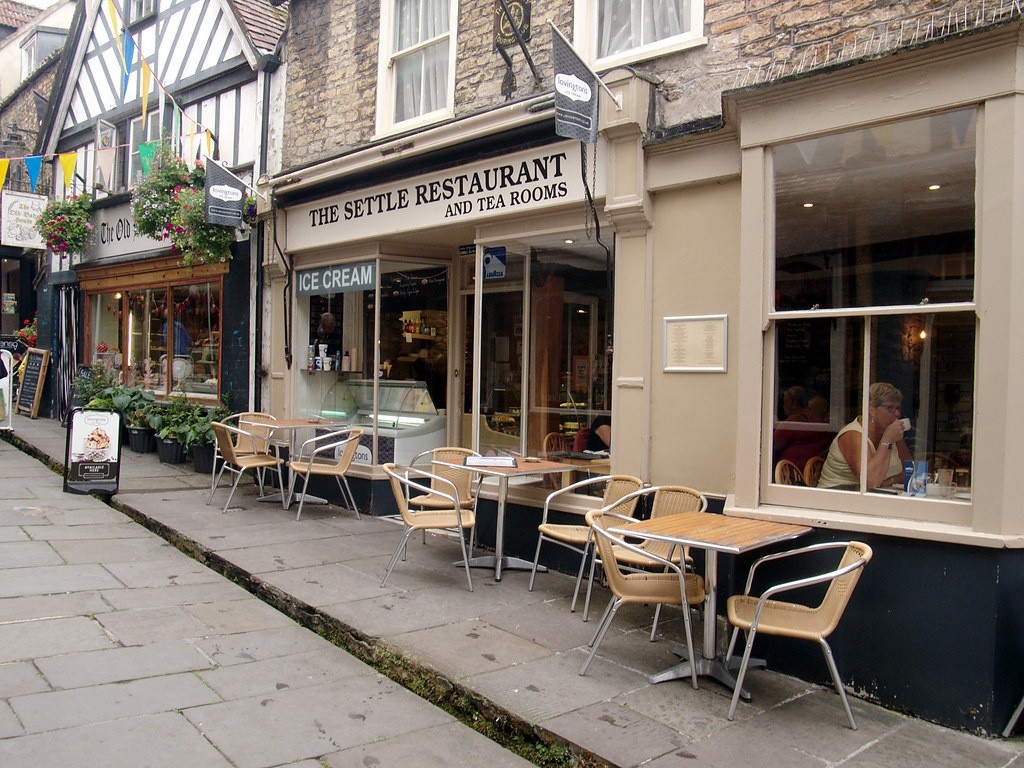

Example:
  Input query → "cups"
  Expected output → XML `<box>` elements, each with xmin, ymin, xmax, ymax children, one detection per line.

<box><xmin>308</xmin><ymin>344</ymin><xmax>333</xmax><ymax>370</ymax></box>
<box><xmin>926</xmin><ymin>483</ymin><xmax>939</xmax><ymax>497</ymax></box>
<box><xmin>956</xmin><ymin>465</ymin><xmax>969</xmax><ymax>487</ymax></box>
<box><xmin>83</xmin><ymin>448</ymin><xmax>110</xmax><ymax>462</ymax></box>
<box><xmin>899</xmin><ymin>418</ymin><xmax>911</xmax><ymax>431</ymax></box>
<box><xmin>939</xmin><ymin>469</ymin><xmax>953</xmax><ymax>500</ymax></box>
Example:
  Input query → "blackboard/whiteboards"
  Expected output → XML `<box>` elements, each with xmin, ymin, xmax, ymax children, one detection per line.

<box><xmin>67</xmin><ymin>363</ymin><xmax>92</xmax><ymax>410</ymax></box>
<box><xmin>16</xmin><ymin>347</ymin><xmax>51</xmax><ymax>412</ymax></box>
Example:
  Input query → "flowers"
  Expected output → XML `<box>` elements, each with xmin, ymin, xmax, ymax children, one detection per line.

<box><xmin>33</xmin><ymin>190</ymin><xmax>98</xmax><ymax>259</ymax></box>
<box><xmin>129</xmin><ymin>127</ymin><xmax>257</xmax><ymax>272</ymax></box>
<box><xmin>13</xmin><ymin>308</ymin><xmax>38</xmax><ymax>347</ymax></box>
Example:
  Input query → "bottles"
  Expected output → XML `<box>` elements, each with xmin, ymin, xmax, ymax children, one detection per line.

<box><xmin>404</xmin><ymin>318</ymin><xmax>437</xmax><ymax>336</ymax></box>
<box><xmin>907</xmin><ymin>460</ymin><xmax>929</xmax><ymax>497</ymax></box>
<box><xmin>336</xmin><ymin>346</ymin><xmax>358</xmax><ymax>372</ymax></box>
<box><xmin>904</xmin><ymin>467</ymin><xmax>913</xmax><ymax>491</ymax></box>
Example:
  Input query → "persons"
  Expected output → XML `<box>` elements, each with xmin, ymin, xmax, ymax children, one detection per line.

<box><xmin>586</xmin><ymin>416</ymin><xmax>611</xmax><ymax>490</ymax></box>
<box><xmin>817</xmin><ymin>382</ymin><xmax>913</xmax><ymax>488</ymax></box>
<box><xmin>773</xmin><ymin>386</ymin><xmax>829</xmax><ymax>480</ymax></box>
<box><xmin>413</xmin><ymin>353</ymin><xmax>447</xmax><ymax>390</ymax></box>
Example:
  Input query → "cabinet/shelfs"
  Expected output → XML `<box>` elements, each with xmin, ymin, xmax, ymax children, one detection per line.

<box><xmin>315</xmin><ymin>380</ymin><xmax>446</xmax><ymax>464</ymax></box>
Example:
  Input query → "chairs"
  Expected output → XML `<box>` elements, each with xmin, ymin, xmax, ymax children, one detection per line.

<box><xmin>380</xmin><ymin>463</ymin><xmax>475</xmax><ymax>592</ymax></box>
<box><xmin>543</xmin><ymin>432</ymin><xmax>573</xmax><ymax>491</ymax></box>
<box><xmin>775</xmin><ymin>460</ymin><xmax>806</xmax><ymax>487</ymax></box>
<box><xmin>583</xmin><ymin>486</ymin><xmax>708</xmax><ymax>642</ymax></box>
<box><xmin>286</xmin><ymin>428</ymin><xmax>363</xmax><ymax>521</ymax></box>
<box><xmin>803</xmin><ymin>457</ymin><xmax>825</xmax><ymax>487</ymax></box>
<box><xmin>401</xmin><ymin>447</ymin><xmax>485</xmax><ymax>561</ymax></box>
<box><xmin>724</xmin><ymin>541</ymin><xmax>873</xmax><ymax>730</ymax></box>
<box><xmin>528</xmin><ymin>475</ymin><xmax>644</xmax><ymax>611</ymax></box>
<box><xmin>579</xmin><ymin>509</ymin><xmax>705</xmax><ymax>690</ymax></box>
<box><xmin>212</xmin><ymin>412</ymin><xmax>277</xmax><ymax>497</ymax></box>
<box><xmin>206</xmin><ymin>421</ymin><xmax>286</xmax><ymax>514</ymax></box>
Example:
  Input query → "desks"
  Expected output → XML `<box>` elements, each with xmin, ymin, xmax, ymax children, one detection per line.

<box><xmin>239</xmin><ymin>418</ymin><xmax>347</xmax><ymax>510</ymax></box>
<box><xmin>430</xmin><ymin>457</ymin><xmax>578</xmax><ymax>580</ymax></box>
<box><xmin>561</xmin><ymin>457</ymin><xmax>611</xmax><ymax>494</ymax></box>
<box><xmin>606</xmin><ymin>510</ymin><xmax>812</xmax><ymax>702</ymax></box>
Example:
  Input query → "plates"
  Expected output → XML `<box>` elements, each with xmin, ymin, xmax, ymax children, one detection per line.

<box><xmin>523</xmin><ymin>457</ymin><xmax>542</xmax><ymax>463</ymax></box>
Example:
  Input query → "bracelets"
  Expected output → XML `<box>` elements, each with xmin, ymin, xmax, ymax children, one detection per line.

<box><xmin>889</xmin><ymin>478</ymin><xmax>895</xmax><ymax>484</ymax></box>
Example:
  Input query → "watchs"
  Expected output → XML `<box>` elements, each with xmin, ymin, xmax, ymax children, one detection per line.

<box><xmin>880</xmin><ymin>443</ymin><xmax>892</xmax><ymax>449</ymax></box>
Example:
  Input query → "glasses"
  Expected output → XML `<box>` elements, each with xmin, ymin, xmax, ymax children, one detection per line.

<box><xmin>875</xmin><ymin>405</ymin><xmax>901</xmax><ymax>413</ymax></box>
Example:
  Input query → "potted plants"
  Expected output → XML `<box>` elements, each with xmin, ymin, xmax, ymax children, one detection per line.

<box><xmin>69</xmin><ymin>359</ymin><xmax>237</xmax><ymax>473</ymax></box>
